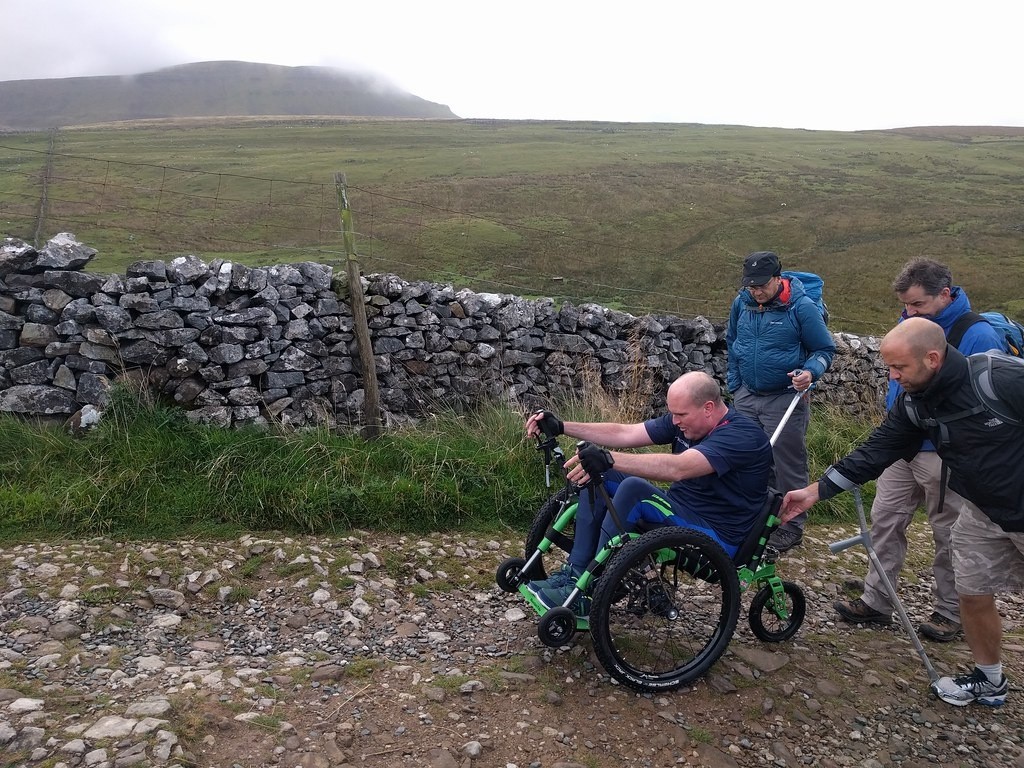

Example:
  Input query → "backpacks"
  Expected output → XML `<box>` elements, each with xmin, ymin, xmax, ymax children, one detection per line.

<box><xmin>946</xmin><ymin>311</ymin><xmax>1024</xmax><ymax>359</ymax></box>
<box><xmin>903</xmin><ymin>348</ymin><xmax>1024</xmax><ymax>512</ymax></box>
<box><xmin>780</xmin><ymin>270</ymin><xmax>829</xmax><ymax>328</ymax></box>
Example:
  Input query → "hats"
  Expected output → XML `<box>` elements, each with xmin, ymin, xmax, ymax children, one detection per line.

<box><xmin>742</xmin><ymin>251</ymin><xmax>781</xmax><ymax>287</ymax></box>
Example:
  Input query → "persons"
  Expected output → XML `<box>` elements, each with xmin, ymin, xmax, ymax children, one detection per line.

<box><xmin>833</xmin><ymin>257</ymin><xmax>1006</xmax><ymax>641</ymax></box>
<box><xmin>772</xmin><ymin>317</ymin><xmax>1024</xmax><ymax>707</ymax></box>
<box><xmin>526</xmin><ymin>371</ymin><xmax>777</xmax><ymax>622</ymax></box>
<box><xmin>727</xmin><ymin>251</ymin><xmax>836</xmax><ymax>552</ymax></box>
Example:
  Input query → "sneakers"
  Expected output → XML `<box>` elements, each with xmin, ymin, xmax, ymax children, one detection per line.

<box><xmin>833</xmin><ymin>598</ymin><xmax>893</xmax><ymax>625</ymax></box>
<box><xmin>919</xmin><ymin>611</ymin><xmax>962</xmax><ymax>642</ymax></box>
<box><xmin>930</xmin><ymin>666</ymin><xmax>1009</xmax><ymax>707</ymax></box>
<box><xmin>526</xmin><ymin>563</ymin><xmax>596</xmax><ymax>621</ymax></box>
<box><xmin>766</xmin><ymin>527</ymin><xmax>804</xmax><ymax>553</ymax></box>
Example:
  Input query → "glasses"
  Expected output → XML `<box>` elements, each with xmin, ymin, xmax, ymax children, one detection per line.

<box><xmin>746</xmin><ymin>277</ymin><xmax>777</xmax><ymax>291</ymax></box>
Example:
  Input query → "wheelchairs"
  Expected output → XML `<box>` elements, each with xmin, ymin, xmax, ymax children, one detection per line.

<box><xmin>495</xmin><ymin>410</ymin><xmax>805</xmax><ymax>696</ymax></box>
